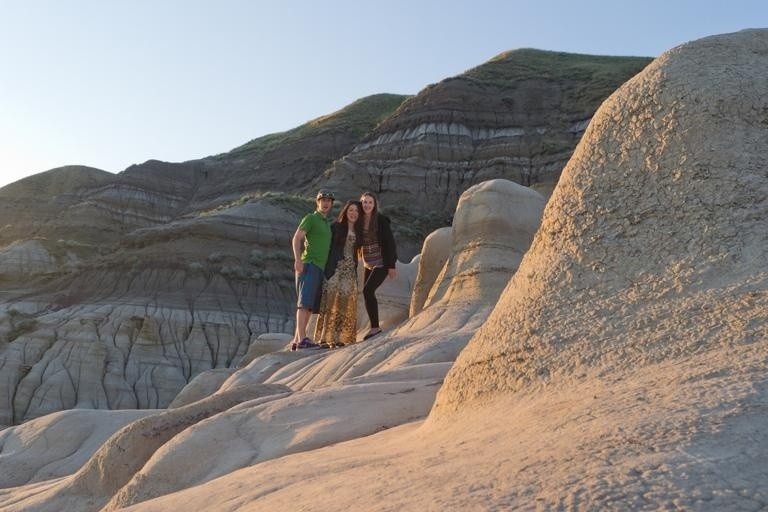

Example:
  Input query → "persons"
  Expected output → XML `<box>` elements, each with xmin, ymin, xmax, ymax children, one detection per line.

<box><xmin>302</xmin><ymin>199</ymin><xmax>364</xmax><ymax>347</ymax></box>
<box><xmin>356</xmin><ymin>192</ymin><xmax>396</xmax><ymax>341</ymax></box>
<box><xmin>291</xmin><ymin>189</ymin><xmax>336</xmax><ymax>351</ymax></box>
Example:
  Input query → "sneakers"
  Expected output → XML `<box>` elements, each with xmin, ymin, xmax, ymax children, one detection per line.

<box><xmin>292</xmin><ymin>337</ymin><xmax>321</xmax><ymax>351</ymax></box>
<box><xmin>364</xmin><ymin>327</ymin><xmax>382</xmax><ymax>340</ymax></box>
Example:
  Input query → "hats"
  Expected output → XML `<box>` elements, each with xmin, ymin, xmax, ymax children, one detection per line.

<box><xmin>317</xmin><ymin>189</ymin><xmax>335</xmax><ymax>201</ymax></box>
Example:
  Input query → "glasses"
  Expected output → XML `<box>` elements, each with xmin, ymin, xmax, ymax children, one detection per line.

<box><xmin>320</xmin><ymin>193</ymin><xmax>335</xmax><ymax>197</ymax></box>
